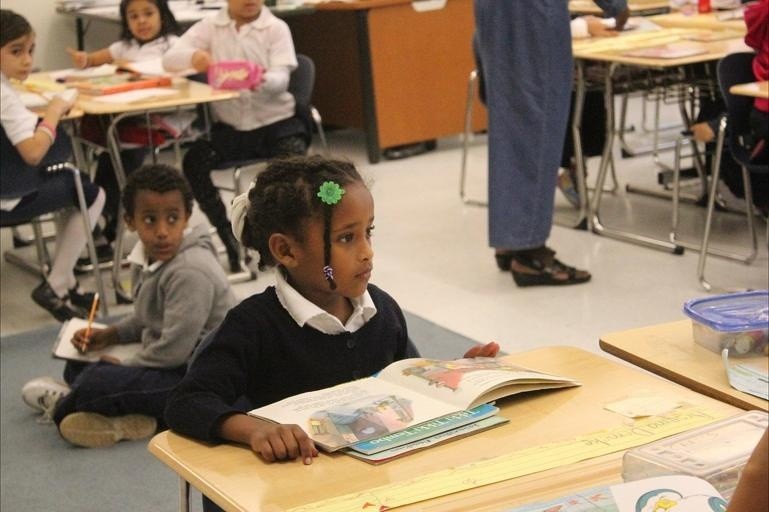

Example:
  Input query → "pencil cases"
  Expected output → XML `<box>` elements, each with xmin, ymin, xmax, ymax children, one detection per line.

<box><xmin>206</xmin><ymin>60</ymin><xmax>264</xmax><ymax>90</ymax></box>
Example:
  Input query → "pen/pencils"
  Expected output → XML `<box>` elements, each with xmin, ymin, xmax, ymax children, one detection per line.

<box><xmin>81</xmin><ymin>292</ymin><xmax>100</xmax><ymax>353</ymax></box>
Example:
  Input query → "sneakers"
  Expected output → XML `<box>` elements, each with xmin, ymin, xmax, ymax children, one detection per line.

<box><xmin>31</xmin><ymin>280</ymin><xmax>100</xmax><ymax>323</ymax></box>
<box><xmin>558</xmin><ymin>172</ymin><xmax>590</xmax><ymax>211</ymax></box>
<box><xmin>59</xmin><ymin>411</ymin><xmax>159</xmax><ymax>448</ymax></box>
<box><xmin>22</xmin><ymin>377</ymin><xmax>73</xmax><ymax>416</ymax></box>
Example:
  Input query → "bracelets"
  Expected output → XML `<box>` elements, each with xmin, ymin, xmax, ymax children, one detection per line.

<box><xmin>81</xmin><ymin>52</ymin><xmax>90</xmax><ymax>69</ymax></box>
<box><xmin>36</xmin><ymin>122</ymin><xmax>57</xmax><ymax>145</ymax></box>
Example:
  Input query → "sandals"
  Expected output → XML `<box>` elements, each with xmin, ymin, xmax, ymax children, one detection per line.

<box><xmin>494</xmin><ymin>246</ymin><xmax>592</xmax><ymax>288</ymax></box>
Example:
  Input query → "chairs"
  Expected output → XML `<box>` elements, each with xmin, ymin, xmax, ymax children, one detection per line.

<box><xmin>184</xmin><ymin>54</ymin><xmax>328</xmax><ymax>263</ymax></box>
<box><xmin>0</xmin><ymin>121</ymin><xmax>111</xmax><ymax>321</ymax></box>
<box><xmin>458</xmin><ymin>30</ymin><xmax>606</xmax><ymax>230</ymax></box>
<box><xmin>698</xmin><ymin>51</ymin><xmax>768</xmax><ymax>292</ymax></box>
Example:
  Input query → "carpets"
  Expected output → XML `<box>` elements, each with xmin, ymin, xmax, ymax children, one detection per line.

<box><xmin>0</xmin><ymin>270</ymin><xmax>508</xmax><ymax>512</ymax></box>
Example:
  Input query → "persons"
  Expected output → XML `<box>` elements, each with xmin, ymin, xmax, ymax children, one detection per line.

<box><xmin>1</xmin><ymin>8</ymin><xmax>108</xmax><ymax>324</ymax></box>
<box><xmin>474</xmin><ymin>14</ymin><xmax>619</xmax><ymax>209</ymax></box>
<box><xmin>20</xmin><ymin>164</ymin><xmax>237</xmax><ymax>449</ymax></box>
<box><xmin>686</xmin><ymin>1</ymin><xmax>769</xmax><ymax>218</ymax></box>
<box><xmin>161</xmin><ymin>0</ymin><xmax>310</xmax><ymax>274</ymax></box>
<box><xmin>63</xmin><ymin>0</ymin><xmax>182</xmax><ymax>272</ymax></box>
<box><xmin>668</xmin><ymin>1</ymin><xmax>742</xmax><ymax>124</ymax></box>
<box><xmin>472</xmin><ymin>1</ymin><xmax>632</xmax><ymax>289</ymax></box>
<box><xmin>162</xmin><ymin>155</ymin><xmax>499</xmax><ymax>511</ymax></box>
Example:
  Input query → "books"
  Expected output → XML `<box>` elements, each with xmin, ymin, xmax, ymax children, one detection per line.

<box><xmin>505</xmin><ymin>475</ymin><xmax>728</xmax><ymax>512</ymax></box>
<box><xmin>717</xmin><ymin>9</ymin><xmax>746</xmax><ymax>22</ymax></box>
<box><xmin>622</xmin><ymin>43</ymin><xmax>710</xmax><ymax>60</ymax></box>
<box><xmin>51</xmin><ymin>317</ymin><xmax>144</xmax><ymax>364</ymax></box>
<box><xmin>680</xmin><ymin>29</ymin><xmax>746</xmax><ymax>43</ymax></box>
<box><xmin>50</xmin><ymin>63</ymin><xmax>190</xmax><ymax>95</ymax></box>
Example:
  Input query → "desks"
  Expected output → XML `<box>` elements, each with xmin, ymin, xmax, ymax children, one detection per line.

<box><xmin>150</xmin><ymin>342</ymin><xmax>750</xmax><ymax>511</ymax></box>
<box><xmin>57</xmin><ymin>1</ymin><xmax>312</xmax><ymax>50</ymax></box>
<box><xmin>598</xmin><ymin>318</ymin><xmax>768</xmax><ymax>415</ymax></box>
<box><xmin>567</xmin><ymin>1</ymin><xmax>756</xmax><ymax>212</ymax></box>
<box><xmin>22</xmin><ymin>61</ymin><xmax>240</xmax><ymax>305</ymax></box>
